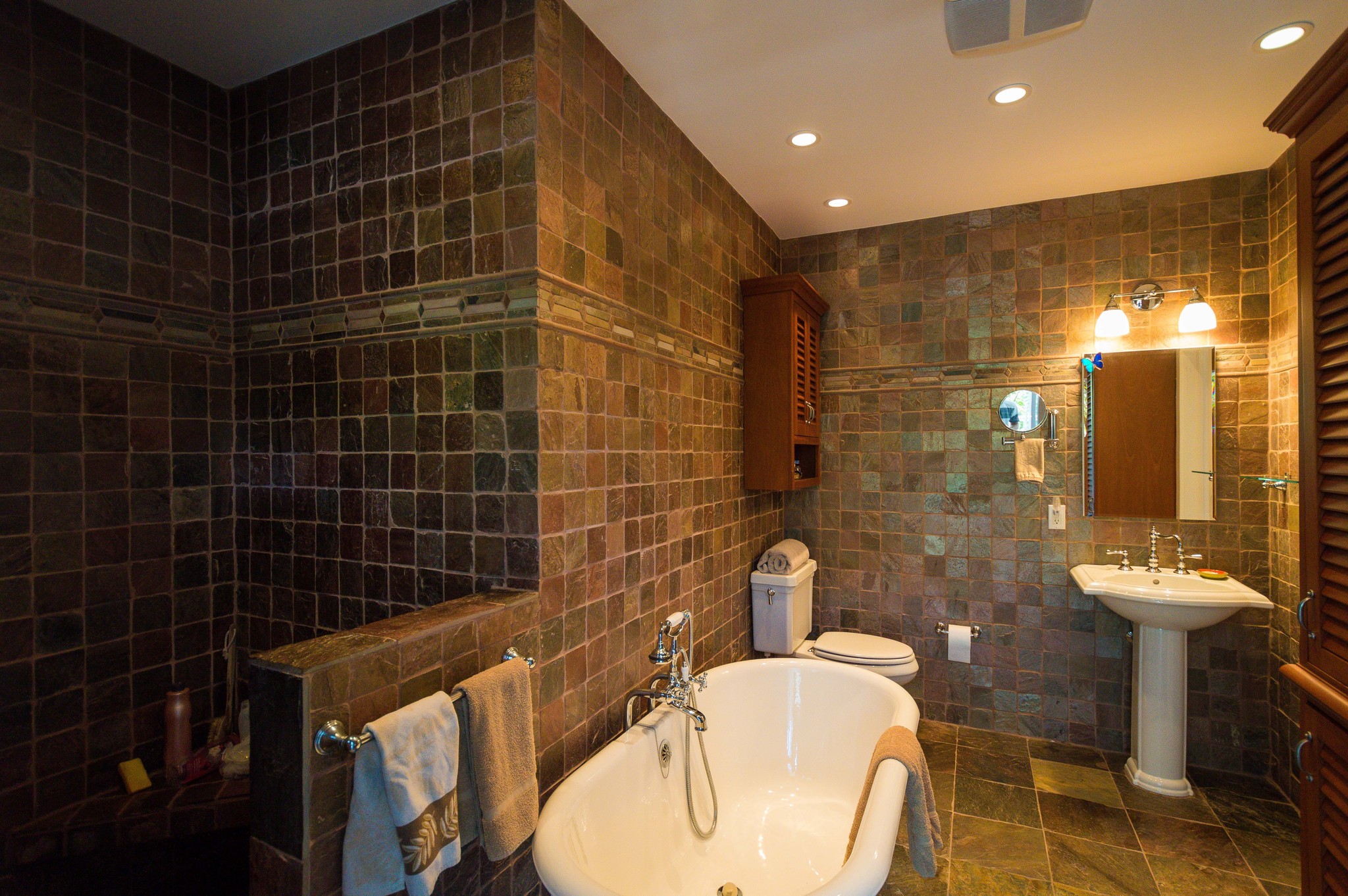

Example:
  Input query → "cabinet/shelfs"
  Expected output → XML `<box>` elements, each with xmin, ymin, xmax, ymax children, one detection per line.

<box><xmin>738</xmin><ymin>271</ymin><xmax>831</xmax><ymax>491</ymax></box>
<box><xmin>1264</xmin><ymin>26</ymin><xmax>1348</xmax><ymax>896</ymax></box>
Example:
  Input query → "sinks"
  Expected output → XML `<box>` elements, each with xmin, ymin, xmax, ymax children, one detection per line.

<box><xmin>1069</xmin><ymin>563</ymin><xmax>1274</xmax><ymax>630</ymax></box>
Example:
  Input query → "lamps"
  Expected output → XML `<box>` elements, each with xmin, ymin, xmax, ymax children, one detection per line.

<box><xmin>1089</xmin><ymin>280</ymin><xmax>1221</xmax><ymax>342</ymax></box>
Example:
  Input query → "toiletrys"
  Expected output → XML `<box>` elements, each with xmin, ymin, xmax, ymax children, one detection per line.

<box><xmin>163</xmin><ymin>681</ymin><xmax>194</xmax><ymax>775</ymax></box>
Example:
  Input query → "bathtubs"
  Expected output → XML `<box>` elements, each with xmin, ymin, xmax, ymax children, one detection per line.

<box><xmin>530</xmin><ymin>657</ymin><xmax>920</xmax><ymax>896</ymax></box>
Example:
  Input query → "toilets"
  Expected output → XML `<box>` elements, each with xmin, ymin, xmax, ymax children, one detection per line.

<box><xmin>750</xmin><ymin>559</ymin><xmax>919</xmax><ymax>686</ymax></box>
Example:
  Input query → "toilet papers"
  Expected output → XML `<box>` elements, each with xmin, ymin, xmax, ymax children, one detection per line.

<box><xmin>947</xmin><ymin>624</ymin><xmax>972</xmax><ymax>664</ymax></box>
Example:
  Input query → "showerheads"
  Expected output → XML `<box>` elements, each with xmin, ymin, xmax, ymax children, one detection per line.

<box><xmin>648</xmin><ymin>609</ymin><xmax>689</xmax><ymax>666</ymax></box>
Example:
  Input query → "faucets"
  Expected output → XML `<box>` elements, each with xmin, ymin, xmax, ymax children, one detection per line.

<box><xmin>623</xmin><ymin>687</ymin><xmax>707</xmax><ymax>736</ymax></box>
<box><xmin>1146</xmin><ymin>526</ymin><xmax>1184</xmax><ymax>573</ymax></box>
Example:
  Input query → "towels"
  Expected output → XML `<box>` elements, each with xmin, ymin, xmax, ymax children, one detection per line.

<box><xmin>452</xmin><ymin>657</ymin><xmax>541</xmax><ymax>862</ymax></box>
<box><xmin>756</xmin><ymin>539</ymin><xmax>810</xmax><ymax>575</ymax></box>
<box><xmin>842</xmin><ymin>725</ymin><xmax>944</xmax><ymax>881</ymax></box>
<box><xmin>1013</xmin><ymin>439</ymin><xmax>1045</xmax><ymax>486</ymax></box>
<box><xmin>341</xmin><ymin>691</ymin><xmax>464</xmax><ymax>896</ymax></box>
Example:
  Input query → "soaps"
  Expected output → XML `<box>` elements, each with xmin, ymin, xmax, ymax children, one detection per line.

<box><xmin>1201</xmin><ymin>571</ymin><xmax>1216</xmax><ymax>575</ymax></box>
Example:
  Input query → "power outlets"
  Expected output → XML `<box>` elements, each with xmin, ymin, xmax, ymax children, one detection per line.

<box><xmin>1045</xmin><ymin>501</ymin><xmax>1070</xmax><ymax>533</ymax></box>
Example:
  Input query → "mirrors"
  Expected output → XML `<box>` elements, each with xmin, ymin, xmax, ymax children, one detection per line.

<box><xmin>1080</xmin><ymin>345</ymin><xmax>1216</xmax><ymax>524</ymax></box>
